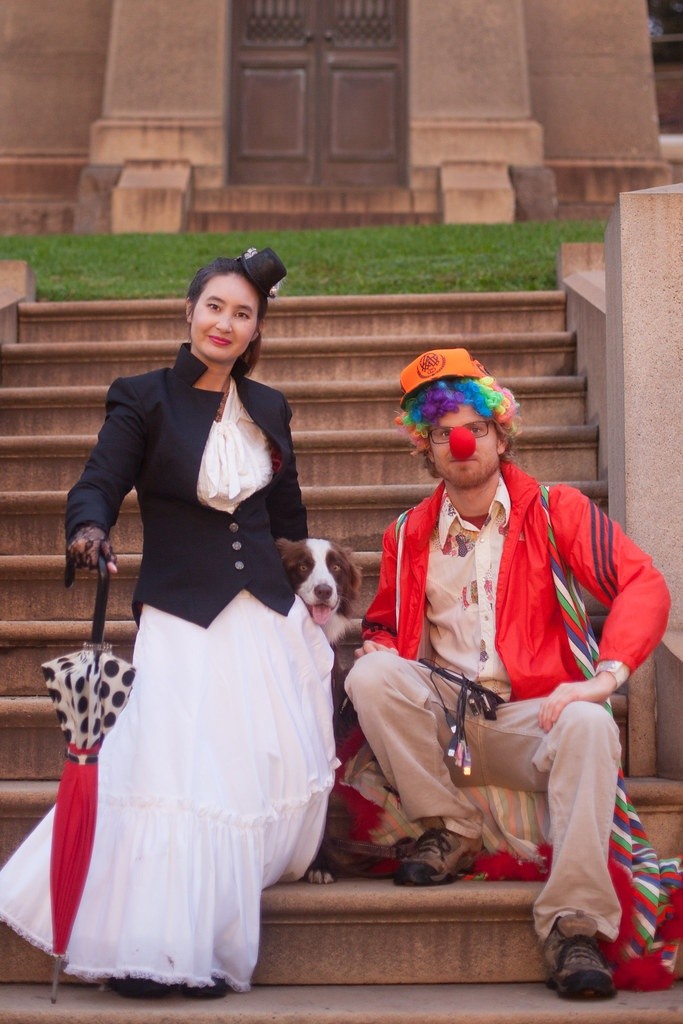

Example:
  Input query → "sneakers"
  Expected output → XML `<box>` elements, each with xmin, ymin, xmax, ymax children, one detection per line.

<box><xmin>544</xmin><ymin>912</ymin><xmax>617</xmax><ymax>1001</ymax></box>
<box><xmin>394</xmin><ymin>816</ymin><xmax>485</xmax><ymax>886</ymax></box>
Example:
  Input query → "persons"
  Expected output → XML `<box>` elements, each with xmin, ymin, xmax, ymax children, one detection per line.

<box><xmin>343</xmin><ymin>346</ymin><xmax>669</xmax><ymax>1001</ymax></box>
<box><xmin>3</xmin><ymin>249</ymin><xmax>336</xmax><ymax>997</ymax></box>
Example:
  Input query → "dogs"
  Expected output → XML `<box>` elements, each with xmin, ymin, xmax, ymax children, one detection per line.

<box><xmin>274</xmin><ymin>534</ymin><xmax>358</xmax><ymax>886</ymax></box>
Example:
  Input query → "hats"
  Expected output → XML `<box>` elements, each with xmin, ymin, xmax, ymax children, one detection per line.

<box><xmin>397</xmin><ymin>347</ymin><xmax>487</xmax><ymax>410</ymax></box>
<box><xmin>242</xmin><ymin>247</ymin><xmax>287</xmax><ymax>298</ymax></box>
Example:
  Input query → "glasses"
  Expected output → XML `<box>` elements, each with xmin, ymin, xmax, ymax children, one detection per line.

<box><xmin>429</xmin><ymin>420</ymin><xmax>498</xmax><ymax>443</ymax></box>
